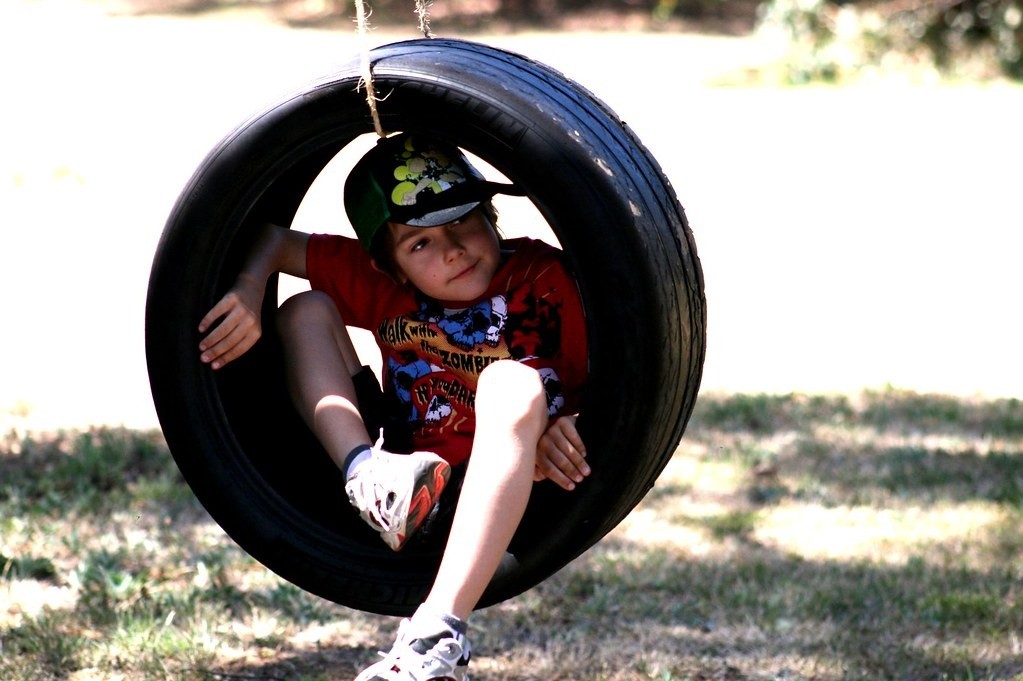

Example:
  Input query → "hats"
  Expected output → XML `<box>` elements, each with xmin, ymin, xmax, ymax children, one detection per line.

<box><xmin>343</xmin><ymin>132</ymin><xmax>531</xmax><ymax>257</ymax></box>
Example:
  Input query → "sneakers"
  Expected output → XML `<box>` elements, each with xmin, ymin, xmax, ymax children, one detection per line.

<box><xmin>344</xmin><ymin>427</ymin><xmax>452</xmax><ymax>553</ymax></box>
<box><xmin>353</xmin><ymin>617</ymin><xmax>473</xmax><ymax>681</ymax></box>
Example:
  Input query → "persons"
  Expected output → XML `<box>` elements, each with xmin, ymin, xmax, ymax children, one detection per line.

<box><xmin>197</xmin><ymin>132</ymin><xmax>592</xmax><ymax>681</ymax></box>
